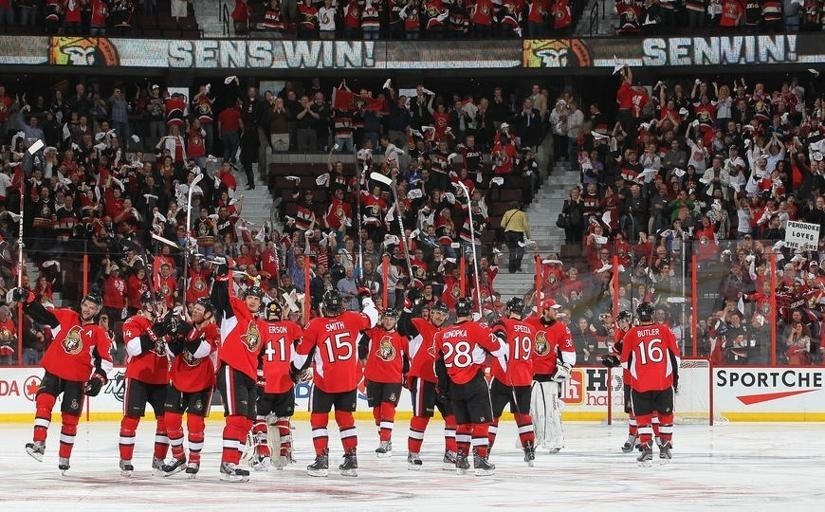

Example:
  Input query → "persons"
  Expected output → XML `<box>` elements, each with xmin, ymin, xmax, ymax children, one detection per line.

<box><xmin>118</xmin><ymin>286</ymin><xmax>576</xmax><ymax>477</ymax></box>
<box><xmin>1</xmin><ymin>1</ymin><xmax>825</xmax><ymax>365</ymax></box>
<box><xmin>6</xmin><ymin>287</ymin><xmax>114</xmax><ymax>477</ymax></box>
<box><xmin>610</xmin><ymin>302</ymin><xmax>681</xmax><ymax>468</ymax></box>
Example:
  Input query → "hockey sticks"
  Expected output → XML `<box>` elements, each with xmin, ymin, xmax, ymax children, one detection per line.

<box><xmin>458</xmin><ymin>178</ymin><xmax>482</xmax><ymax>319</ymax></box>
<box><xmin>17</xmin><ymin>139</ymin><xmax>44</xmax><ymax>288</ymax></box>
<box><xmin>370</xmin><ymin>171</ymin><xmax>418</xmax><ymax>304</ymax></box>
<box><xmin>183</xmin><ymin>172</ymin><xmax>203</xmax><ymax>315</ymax></box>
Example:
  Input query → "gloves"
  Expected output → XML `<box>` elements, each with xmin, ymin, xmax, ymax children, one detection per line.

<box><xmin>288</xmin><ymin>362</ymin><xmax>301</xmax><ymax>384</ymax></box>
<box><xmin>176</xmin><ymin>320</ymin><xmax>191</xmax><ymax>341</ymax></box>
<box><xmin>7</xmin><ymin>286</ymin><xmax>34</xmax><ymax>304</ymax></box>
<box><xmin>612</xmin><ymin>342</ymin><xmax>622</xmax><ymax>354</ymax></box>
<box><xmin>146</xmin><ymin>321</ymin><xmax>172</xmax><ymax>342</ymax></box>
<box><xmin>492</xmin><ymin>320</ymin><xmax>507</xmax><ymax>334</ymax></box>
<box><xmin>84</xmin><ymin>370</ymin><xmax>108</xmax><ymax>396</ymax></box>
<box><xmin>602</xmin><ymin>357</ymin><xmax>620</xmax><ymax>367</ymax></box>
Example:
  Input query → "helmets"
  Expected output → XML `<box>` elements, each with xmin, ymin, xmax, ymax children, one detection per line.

<box><xmin>506</xmin><ymin>297</ymin><xmax>524</xmax><ymax>315</ymax></box>
<box><xmin>382</xmin><ymin>308</ymin><xmax>399</xmax><ymax>318</ymax></box>
<box><xmin>80</xmin><ymin>292</ymin><xmax>103</xmax><ymax>315</ymax></box>
<box><xmin>432</xmin><ymin>301</ymin><xmax>449</xmax><ymax>315</ymax></box>
<box><xmin>193</xmin><ymin>297</ymin><xmax>216</xmax><ymax>319</ymax></box>
<box><xmin>455</xmin><ymin>297</ymin><xmax>474</xmax><ymax>317</ymax></box>
<box><xmin>322</xmin><ymin>289</ymin><xmax>342</xmax><ymax>313</ymax></box>
<box><xmin>637</xmin><ymin>301</ymin><xmax>656</xmax><ymax>318</ymax></box>
<box><xmin>140</xmin><ymin>290</ymin><xmax>166</xmax><ymax>310</ymax></box>
<box><xmin>616</xmin><ymin>309</ymin><xmax>638</xmax><ymax>329</ymax></box>
<box><xmin>403</xmin><ymin>288</ymin><xmax>426</xmax><ymax>313</ymax></box>
<box><xmin>243</xmin><ymin>286</ymin><xmax>281</xmax><ymax>316</ymax></box>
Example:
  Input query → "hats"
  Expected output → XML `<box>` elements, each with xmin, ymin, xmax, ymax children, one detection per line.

<box><xmin>542</xmin><ymin>298</ymin><xmax>561</xmax><ymax>310</ymax></box>
<box><xmin>209</xmin><ymin>256</ymin><xmax>227</xmax><ymax>265</ymax></box>
<box><xmin>111</xmin><ymin>264</ymin><xmax>119</xmax><ymax>271</ymax></box>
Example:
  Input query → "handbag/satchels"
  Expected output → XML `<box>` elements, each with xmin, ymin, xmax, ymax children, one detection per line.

<box><xmin>556</xmin><ymin>213</ymin><xmax>572</xmax><ymax>229</ymax></box>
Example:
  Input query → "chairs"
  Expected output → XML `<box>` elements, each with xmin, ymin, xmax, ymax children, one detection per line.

<box><xmin>0</xmin><ymin>1</ymin><xmax>825</xmax><ymax>372</ymax></box>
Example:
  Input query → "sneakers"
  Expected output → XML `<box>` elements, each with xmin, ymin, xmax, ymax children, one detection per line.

<box><xmin>338</xmin><ymin>455</ymin><xmax>358</xmax><ymax>470</ymax></box>
<box><xmin>406</xmin><ymin>452</ymin><xmax>422</xmax><ymax>465</ymax></box>
<box><xmin>509</xmin><ymin>267</ymin><xmax>523</xmax><ymax>274</ymax></box>
<box><xmin>59</xmin><ymin>456</ymin><xmax>71</xmax><ymax>470</ymax></box>
<box><xmin>307</xmin><ymin>455</ymin><xmax>330</xmax><ymax>471</ymax></box>
<box><xmin>442</xmin><ymin>443</ymin><xmax>565</xmax><ymax>471</ymax></box>
<box><xmin>375</xmin><ymin>442</ymin><xmax>393</xmax><ymax>452</ymax></box>
<box><xmin>185</xmin><ymin>462</ymin><xmax>200</xmax><ymax>475</ymax></box>
<box><xmin>152</xmin><ymin>457</ymin><xmax>165</xmax><ymax>471</ymax></box>
<box><xmin>25</xmin><ymin>441</ymin><xmax>46</xmax><ymax>456</ymax></box>
<box><xmin>217</xmin><ymin>456</ymin><xmax>290</xmax><ymax>478</ymax></box>
<box><xmin>162</xmin><ymin>459</ymin><xmax>186</xmax><ymax>473</ymax></box>
<box><xmin>622</xmin><ymin>434</ymin><xmax>673</xmax><ymax>462</ymax></box>
<box><xmin>119</xmin><ymin>460</ymin><xmax>133</xmax><ymax>471</ymax></box>
